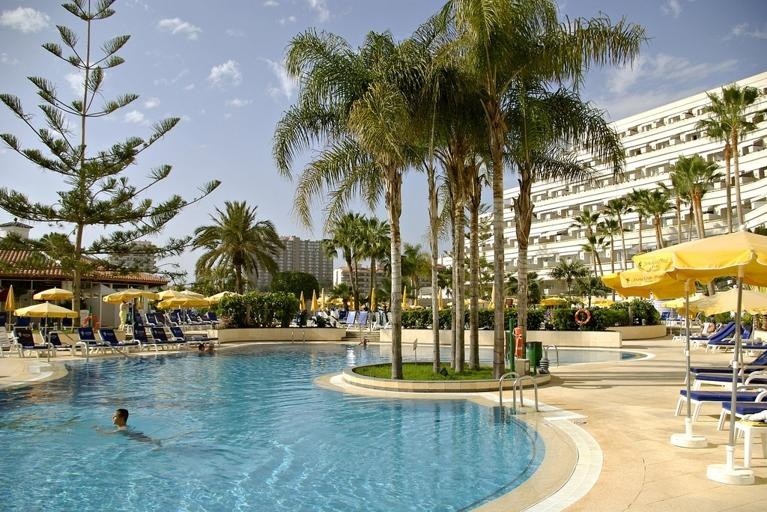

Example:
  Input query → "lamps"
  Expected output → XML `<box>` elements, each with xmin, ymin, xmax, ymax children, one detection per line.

<box><xmin>676</xmin><ymin>320</ymin><xmax>767</xmax><ymax>468</ymax></box>
<box><xmin>0</xmin><ymin>306</ymin><xmax>219</xmax><ymax>358</ymax></box>
<box><xmin>338</xmin><ymin>311</ymin><xmax>392</xmax><ymax>330</ymax></box>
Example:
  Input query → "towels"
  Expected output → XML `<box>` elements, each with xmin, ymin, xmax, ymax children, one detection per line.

<box><xmin>525</xmin><ymin>342</ymin><xmax>542</xmax><ymax>376</ymax></box>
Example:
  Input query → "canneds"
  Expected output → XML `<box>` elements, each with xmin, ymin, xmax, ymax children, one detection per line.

<box><xmin>575</xmin><ymin>308</ymin><xmax>591</xmax><ymax>327</ymax></box>
<box><xmin>82</xmin><ymin>315</ymin><xmax>99</xmax><ymax>333</ymax></box>
<box><xmin>512</xmin><ymin>326</ymin><xmax>525</xmax><ymax>359</ymax></box>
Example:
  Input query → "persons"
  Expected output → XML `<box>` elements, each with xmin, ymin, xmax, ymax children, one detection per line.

<box><xmin>205</xmin><ymin>342</ymin><xmax>216</xmax><ymax>352</ymax></box>
<box><xmin>198</xmin><ymin>344</ymin><xmax>206</xmax><ymax>352</ymax></box>
<box><xmin>117</xmin><ymin>300</ymin><xmax>134</xmax><ymax>330</ymax></box>
<box><xmin>300</xmin><ymin>308</ymin><xmax>307</xmax><ymax>322</ymax></box>
<box><xmin>357</xmin><ymin>337</ymin><xmax>368</xmax><ymax>345</ymax></box>
<box><xmin>94</xmin><ymin>407</ymin><xmax>194</xmax><ymax>446</ymax></box>
<box><xmin>701</xmin><ymin>319</ymin><xmax>715</xmax><ymax>338</ymax></box>
<box><xmin>721</xmin><ymin>324</ymin><xmax>744</xmax><ymax>344</ymax></box>
<box><xmin>330</xmin><ymin>308</ymin><xmax>340</xmax><ymax>321</ymax></box>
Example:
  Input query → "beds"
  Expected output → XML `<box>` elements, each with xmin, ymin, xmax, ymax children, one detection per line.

<box><xmin>298</xmin><ymin>283</ymin><xmax>495</xmax><ymax>312</ymax></box>
<box><xmin>682</xmin><ymin>285</ymin><xmax>767</xmax><ymax>392</ymax></box>
<box><xmin>32</xmin><ymin>285</ymin><xmax>74</xmax><ymax>305</ymax></box>
<box><xmin>601</xmin><ymin>261</ymin><xmax>719</xmax><ymax>433</ymax></box>
<box><xmin>102</xmin><ymin>285</ymin><xmax>158</xmax><ymax>345</ymax></box>
<box><xmin>147</xmin><ymin>289</ymin><xmax>246</xmax><ymax>331</ymax></box>
<box><xmin>662</xmin><ymin>292</ymin><xmax>706</xmax><ymax>375</ymax></box>
<box><xmin>583</xmin><ymin>299</ymin><xmax>617</xmax><ymax>306</ymax></box>
<box><xmin>12</xmin><ymin>301</ymin><xmax>79</xmax><ymax>346</ymax></box>
<box><xmin>4</xmin><ymin>283</ymin><xmax>15</xmax><ymax>331</ymax></box>
<box><xmin>631</xmin><ymin>227</ymin><xmax>767</xmax><ymax>466</ymax></box>
<box><xmin>538</xmin><ymin>297</ymin><xmax>568</xmax><ymax>307</ymax></box>
